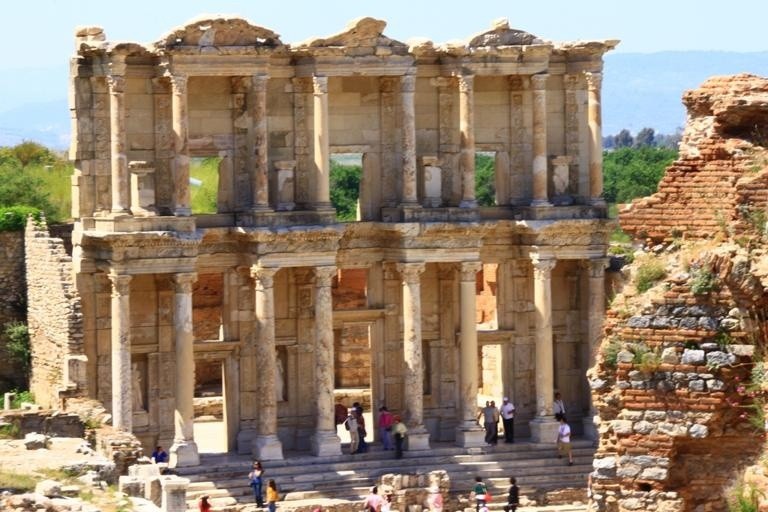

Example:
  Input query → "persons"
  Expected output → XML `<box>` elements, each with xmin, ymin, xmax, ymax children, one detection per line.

<box><xmin>266</xmin><ymin>478</ymin><xmax>280</xmax><ymax>512</ymax></box>
<box><xmin>469</xmin><ymin>476</ymin><xmax>488</xmax><ymax>512</ymax></box>
<box><xmin>477</xmin><ymin>396</ymin><xmax>516</xmax><ymax>444</ymax></box>
<box><xmin>363</xmin><ymin>486</ymin><xmax>385</xmax><ymax>512</ymax></box>
<box><xmin>151</xmin><ymin>445</ymin><xmax>172</xmax><ymax>475</ymax></box>
<box><xmin>345</xmin><ymin>401</ymin><xmax>408</xmax><ymax>459</ymax></box>
<box><xmin>427</xmin><ymin>485</ymin><xmax>444</xmax><ymax>512</ymax></box>
<box><xmin>379</xmin><ymin>495</ymin><xmax>392</xmax><ymax>512</ymax></box>
<box><xmin>556</xmin><ymin>415</ymin><xmax>574</xmax><ymax>466</ymax></box>
<box><xmin>248</xmin><ymin>460</ymin><xmax>266</xmax><ymax>508</ymax></box>
<box><xmin>554</xmin><ymin>392</ymin><xmax>566</xmax><ymax>421</ymax></box>
<box><xmin>478</xmin><ymin>502</ymin><xmax>489</xmax><ymax>512</ymax></box>
<box><xmin>503</xmin><ymin>477</ymin><xmax>521</xmax><ymax>512</ymax></box>
<box><xmin>198</xmin><ymin>494</ymin><xmax>212</xmax><ymax>512</ymax></box>
